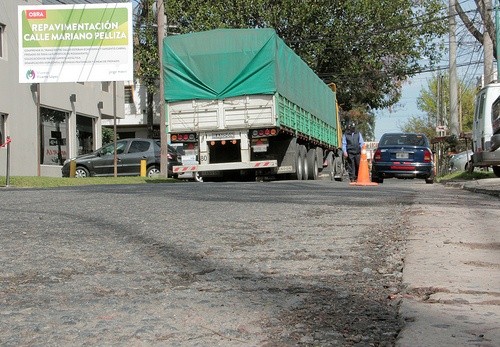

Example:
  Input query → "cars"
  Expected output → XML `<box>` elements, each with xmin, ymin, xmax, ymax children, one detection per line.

<box><xmin>371</xmin><ymin>132</ymin><xmax>435</xmax><ymax>184</ymax></box>
<box><xmin>448</xmin><ymin>148</ymin><xmax>475</xmax><ymax>174</ymax></box>
<box><xmin>61</xmin><ymin>138</ymin><xmax>183</xmax><ymax>178</ymax></box>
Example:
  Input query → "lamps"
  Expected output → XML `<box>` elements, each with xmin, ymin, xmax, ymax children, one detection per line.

<box><xmin>97</xmin><ymin>101</ymin><xmax>103</xmax><ymax>109</ymax></box>
<box><xmin>30</xmin><ymin>83</ymin><xmax>37</xmax><ymax>92</ymax></box>
<box><xmin>70</xmin><ymin>94</ymin><xmax>76</xmax><ymax>102</ymax></box>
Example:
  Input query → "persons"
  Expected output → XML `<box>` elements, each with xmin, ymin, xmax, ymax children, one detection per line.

<box><xmin>341</xmin><ymin>123</ymin><xmax>366</xmax><ymax>182</ymax></box>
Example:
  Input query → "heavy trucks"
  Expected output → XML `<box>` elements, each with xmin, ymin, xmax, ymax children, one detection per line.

<box><xmin>162</xmin><ymin>28</ymin><xmax>346</xmax><ymax>182</ymax></box>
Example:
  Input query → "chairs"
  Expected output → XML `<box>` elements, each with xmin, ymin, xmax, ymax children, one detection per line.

<box><xmin>387</xmin><ymin>136</ymin><xmax>398</xmax><ymax>144</ymax></box>
<box><xmin>405</xmin><ymin>134</ymin><xmax>418</xmax><ymax>144</ymax></box>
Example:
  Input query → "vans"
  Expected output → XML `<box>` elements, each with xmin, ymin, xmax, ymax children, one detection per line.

<box><xmin>469</xmin><ymin>82</ymin><xmax>500</xmax><ymax>177</ymax></box>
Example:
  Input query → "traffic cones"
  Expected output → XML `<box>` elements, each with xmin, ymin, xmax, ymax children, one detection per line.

<box><xmin>348</xmin><ymin>145</ymin><xmax>379</xmax><ymax>186</ymax></box>
